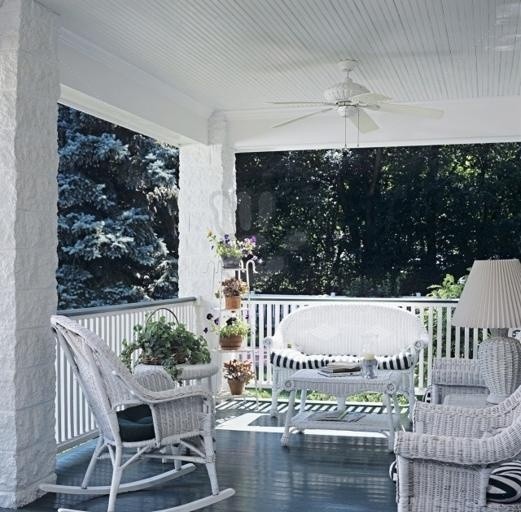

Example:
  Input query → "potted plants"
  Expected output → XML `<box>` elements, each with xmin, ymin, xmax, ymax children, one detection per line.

<box><xmin>220</xmin><ymin>358</ymin><xmax>255</xmax><ymax>395</ymax></box>
<box><xmin>118</xmin><ymin>307</ymin><xmax>208</xmax><ymax>383</ymax></box>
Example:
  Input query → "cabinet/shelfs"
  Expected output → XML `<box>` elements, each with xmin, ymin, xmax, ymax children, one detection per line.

<box><xmin>202</xmin><ymin>257</ymin><xmax>261</xmax><ymax>413</ymax></box>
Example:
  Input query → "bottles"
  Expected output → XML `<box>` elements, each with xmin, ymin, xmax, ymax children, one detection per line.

<box><xmin>360</xmin><ymin>352</ymin><xmax>380</xmax><ymax>380</ymax></box>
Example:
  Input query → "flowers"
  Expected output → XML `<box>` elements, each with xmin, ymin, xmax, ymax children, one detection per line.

<box><xmin>212</xmin><ymin>277</ymin><xmax>248</xmax><ymax>297</ymax></box>
<box><xmin>204</xmin><ymin>312</ymin><xmax>251</xmax><ymax>335</ymax></box>
<box><xmin>204</xmin><ymin>226</ymin><xmax>257</xmax><ymax>258</ymax></box>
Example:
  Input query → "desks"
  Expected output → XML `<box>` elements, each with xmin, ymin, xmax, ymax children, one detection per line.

<box><xmin>135</xmin><ymin>351</ymin><xmax>215</xmax><ymax>453</ymax></box>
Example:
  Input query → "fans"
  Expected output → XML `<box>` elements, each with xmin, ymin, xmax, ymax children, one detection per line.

<box><xmin>259</xmin><ymin>57</ymin><xmax>446</xmax><ymax>149</ymax></box>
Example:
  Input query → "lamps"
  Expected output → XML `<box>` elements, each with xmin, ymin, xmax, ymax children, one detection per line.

<box><xmin>334</xmin><ymin>103</ymin><xmax>358</xmax><ymax>118</ymax></box>
<box><xmin>448</xmin><ymin>257</ymin><xmax>521</xmax><ymax>404</ymax></box>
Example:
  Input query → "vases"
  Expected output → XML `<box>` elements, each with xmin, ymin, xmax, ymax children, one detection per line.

<box><xmin>219</xmin><ymin>333</ymin><xmax>243</xmax><ymax>351</ymax></box>
<box><xmin>219</xmin><ymin>253</ymin><xmax>241</xmax><ymax>268</ymax></box>
<box><xmin>224</xmin><ymin>295</ymin><xmax>241</xmax><ymax>310</ymax></box>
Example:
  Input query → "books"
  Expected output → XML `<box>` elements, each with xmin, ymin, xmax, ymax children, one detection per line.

<box><xmin>318</xmin><ymin>370</ymin><xmax>361</xmax><ymax>377</ymax></box>
<box><xmin>323</xmin><ymin>365</ymin><xmax>361</xmax><ymax>373</ymax></box>
<box><xmin>325</xmin><ymin>410</ymin><xmax>349</xmax><ymax>421</ymax></box>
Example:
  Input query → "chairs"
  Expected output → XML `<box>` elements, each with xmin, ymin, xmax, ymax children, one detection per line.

<box><xmin>391</xmin><ymin>355</ymin><xmax>521</xmax><ymax>512</ymax></box>
<box><xmin>37</xmin><ymin>314</ymin><xmax>235</xmax><ymax>511</ymax></box>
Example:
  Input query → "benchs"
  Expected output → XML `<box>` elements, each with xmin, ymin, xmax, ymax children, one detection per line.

<box><xmin>262</xmin><ymin>301</ymin><xmax>429</xmax><ymax>419</ymax></box>
